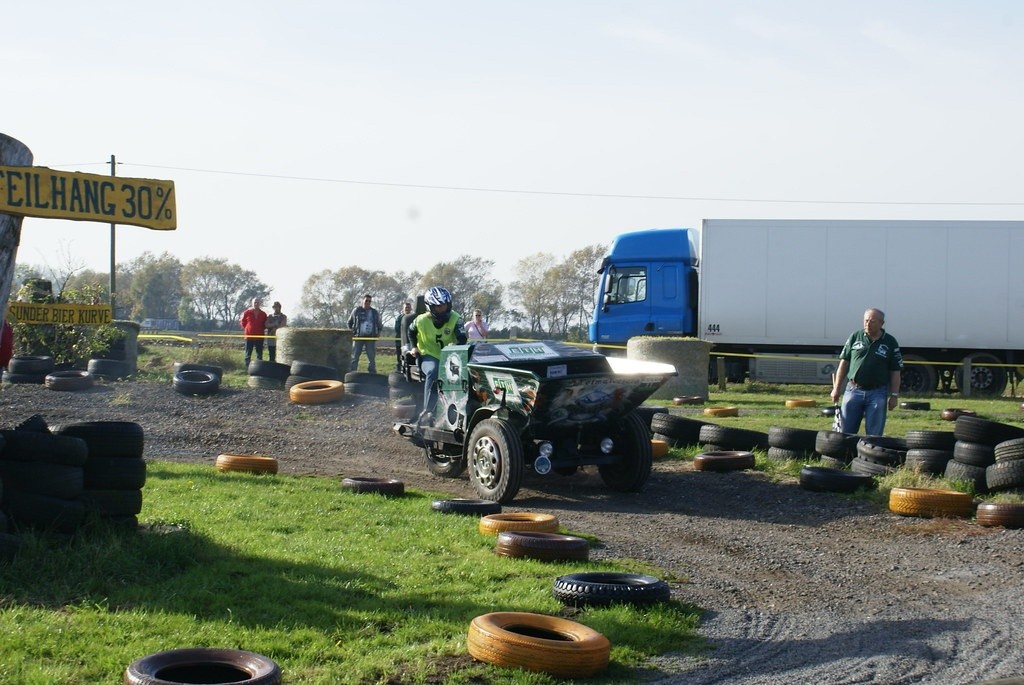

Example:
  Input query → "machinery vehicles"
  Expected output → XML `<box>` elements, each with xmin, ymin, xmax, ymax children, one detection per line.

<box><xmin>394</xmin><ymin>340</ymin><xmax>679</xmax><ymax>505</ymax></box>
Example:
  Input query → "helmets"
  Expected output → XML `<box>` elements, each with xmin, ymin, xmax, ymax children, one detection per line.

<box><xmin>424</xmin><ymin>287</ymin><xmax>452</xmax><ymax>323</ymax></box>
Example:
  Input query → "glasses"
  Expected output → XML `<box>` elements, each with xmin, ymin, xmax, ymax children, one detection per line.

<box><xmin>364</xmin><ymin>300</ymin><xmax>371</xmax><ymax>303</ymax></box>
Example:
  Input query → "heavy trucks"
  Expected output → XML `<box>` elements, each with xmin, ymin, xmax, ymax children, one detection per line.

<box><xmin>588</xmin><ymin>218</ymin><xmax>1023</xmax><ymax>404</ymax></box>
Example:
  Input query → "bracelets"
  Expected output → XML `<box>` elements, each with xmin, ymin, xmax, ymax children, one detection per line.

<box><xmin>891</xmin><ymin>393</ymin><xmax>899</xmax><ymax>396</ymax></box>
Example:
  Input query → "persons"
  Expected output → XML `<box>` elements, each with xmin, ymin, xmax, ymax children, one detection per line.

<box><xmin>830</xmin><ymin>308</ymin><xmax>904</xmax><ymax>434</ymax></box>
<box><xmin>239</xmin><ymin>297</ymin><xmax>268</xmax><ymax>370</ymax></box>
<box><xmin>265</xmin><ymin>302</ymin><xmax>288</xmax><ymax>360</ymax></box>
<box><xmin>464</xmin><ymin>308</ymin><xmax>489</xmax><ymax>340</ymax></box>
<box><xmin>346</xmin><ymin>294</ymin><xmax>384</xmax><ymax>371</ymax></box>
<box><xmin>394</xmin><ymin>303</ymin><xmax>412</xmax><ymax>374</ymax></box>
<box><xmin>406</xmin><ymin>286</ymin><xmax>469</xmax><ymax>422</ymax></box>
